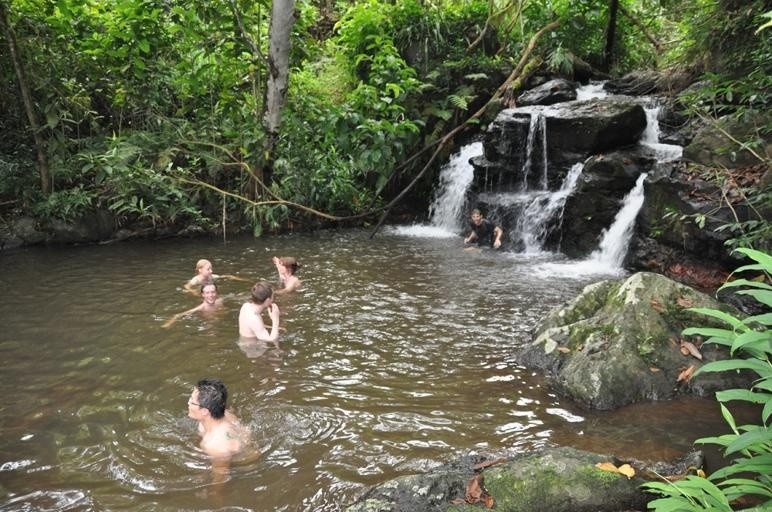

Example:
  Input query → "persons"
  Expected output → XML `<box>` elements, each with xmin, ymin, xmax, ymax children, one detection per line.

<box><xmin>269</xmin><ymin>254</ymin><xmax>303</xmax><ymax>295</ymax></box>
<box><xmin>238</xmin><ymin>281</ymin><xmax>281</xmax><ymax>342</ymax></box>
<box><xmin>462</xmin><ymin>209</ymin><xmax>504</xmax><ymax>255</ymax></box>
<box><xmin>186</xmin><ymin>378</ymin><xmax>251</xmax><ymax>487</ymax></box>
<box><xmin>161</xmin><ymin>280</ymin><xmax>236</xmax><ymax>330</ymax></box>
<box><xmin>183</xmin><ymin>257</ymin><xmax>245</xmax><ymax>296</ymax></box>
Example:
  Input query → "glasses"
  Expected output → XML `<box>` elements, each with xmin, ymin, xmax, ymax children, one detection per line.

<box><xmin>188</xmin><ymin>398</ymin><xmax>206</xmax><ymax>408</ymax></box>
